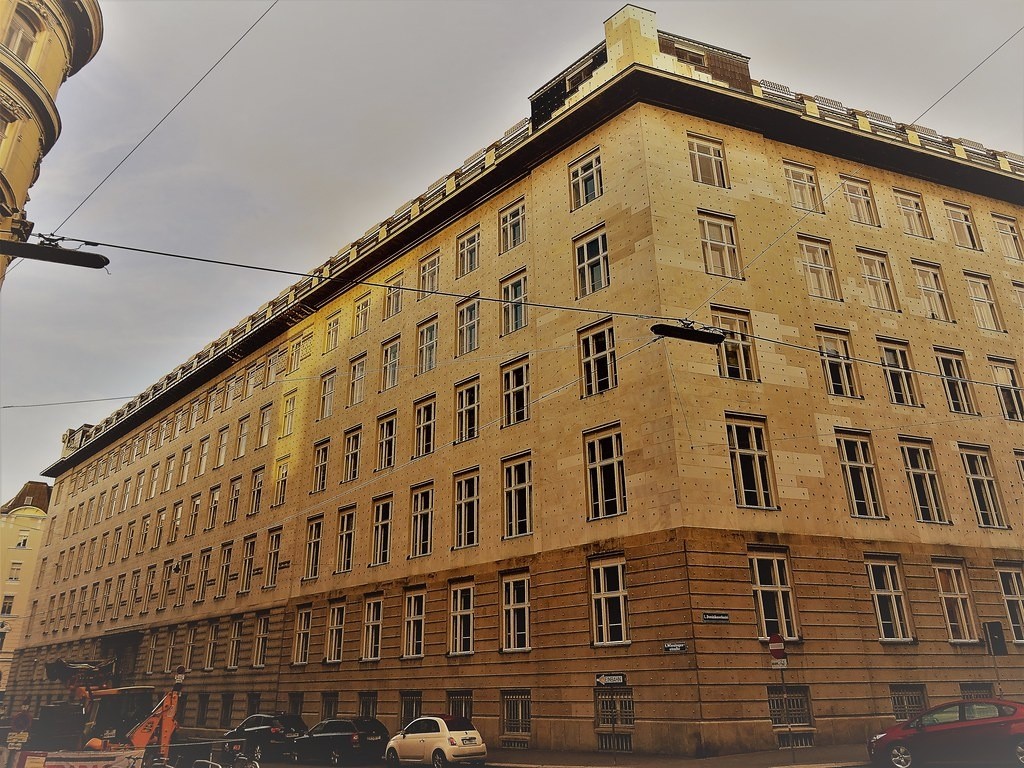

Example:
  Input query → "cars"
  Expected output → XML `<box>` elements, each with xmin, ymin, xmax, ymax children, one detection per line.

<box><xmin>385</xmin><ymin>715</ymin><xmax>488</xmax><ymax>768</ymax></box>
<box><xmin>867</xmin><ymin>698</ymin><xmax>1024</xmax><ymax>768</ymax></box>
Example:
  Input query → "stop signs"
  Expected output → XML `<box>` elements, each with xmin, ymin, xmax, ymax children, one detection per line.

<box><xmin>768</xmin><ymin>633</ymin><xmax>786</xmax><ymax>659</ymax></box>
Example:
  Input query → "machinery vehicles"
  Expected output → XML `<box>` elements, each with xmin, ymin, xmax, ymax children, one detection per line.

<box><xmin>3</xmin><ymin>657</ymin><xmax>220</xmax><ymax>768</ymax></box>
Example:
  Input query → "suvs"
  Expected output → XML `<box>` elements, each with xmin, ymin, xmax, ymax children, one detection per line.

<box><xmin>223</xmin><ymin>711</ymin><xmax>309</xmax><ymax>764</ymax></box>
<box><xmin>293</xmin><ymin>717</ymin><xmax>390</xmax><ymax>768</ymax></box>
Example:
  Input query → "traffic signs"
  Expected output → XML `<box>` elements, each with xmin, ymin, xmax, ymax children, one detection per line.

<box><xmin>594</xmin><ymin>674</ymin><xmax>626</xmax><ymax>688</ymax></box>
